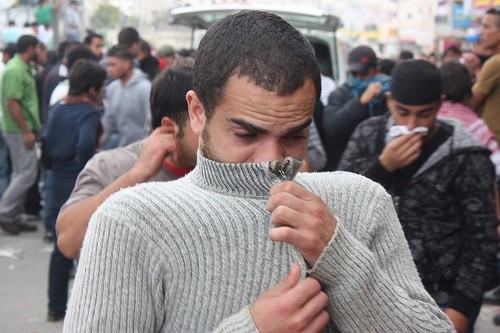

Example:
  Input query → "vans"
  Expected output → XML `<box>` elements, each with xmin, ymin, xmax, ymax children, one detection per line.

<box><xmin>170</xmin><ymin>6</ymin><xmax>344</xmax><ymax>87</ymax></box>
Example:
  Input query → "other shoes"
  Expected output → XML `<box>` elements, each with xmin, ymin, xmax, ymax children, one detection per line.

<box><xmin>46</xmin><ymin>309</ymin><xmax>66</xmax><ymax>322</ymax></box>
<box><xmin>15</xmin><ymin>222</ymin><xmax>37</xmax><ymax>232</ymax></box>
<box><xmin>1</xmin><ymin>219</ymin><xmax>20</xmax><ymax>235</ymax></box>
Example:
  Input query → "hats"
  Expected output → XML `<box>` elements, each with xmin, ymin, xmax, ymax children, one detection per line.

<box><xmin>390</xmin><ymin>58</ymin><xmax>441</xmax><ymax>105</ymax></box>
<box><xmin>345</xmin><ymin>46</ymin><xmax>378</xmax><ymax>74</ymax></box>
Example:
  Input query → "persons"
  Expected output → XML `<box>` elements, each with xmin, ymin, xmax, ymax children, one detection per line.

<box><xmin>55</xmin><ymin>63</ymin><xmax>312</xmax><ymax>260</ymax></box>
<box><xmin>61</xmin><ymin>10</ymin><xmax>458</xmax><ymax>333</ymax></box>
<box><xmin>317</xmin><ymin>43</ymin><xmax>393</xmax><ymax>171</ymax></box>
<box><xmin>1</xmin><ymin>27</ymin><xmax>199</xmax><ymax>222</ymax></box>
<box><xmin>372</xmin><ymin>7</ymin><xmax>500</xmax><ymax>327</ymax></box>
<box><xmin>335</xmin><ymin>58</ymin><xmax>499</xmax><ymax>333</ymax></box>
<box><xmin>1</xmin><ymin>35</ymin><xmax>45</xmax><ymax>236</ymax></box>
<box><xmin>39</xmin><ymin>60</ymin><xmax>109</xmax><ymax>322</ymax></box>
